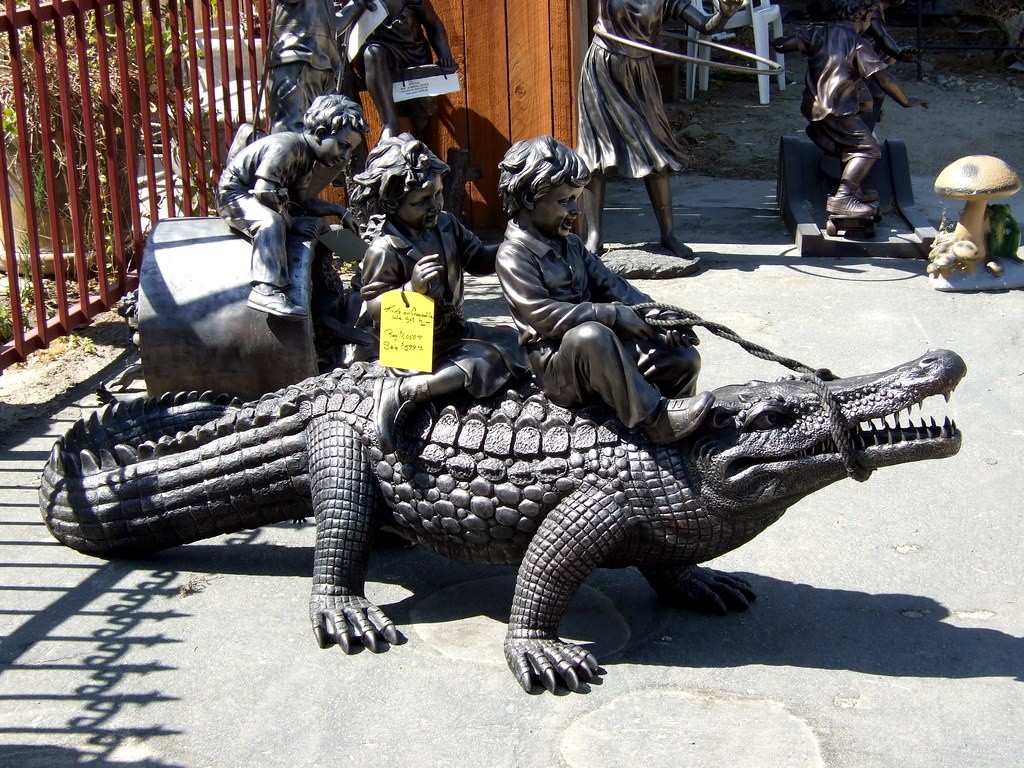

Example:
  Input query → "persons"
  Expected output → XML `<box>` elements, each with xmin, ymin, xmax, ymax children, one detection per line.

<box><xmin>348</xmin><ymin>131</ymin><xmax>534</xmax><ymax>455</ymax></box>
<box><xmin>859</xmin><ymin>0</ymin><xmax>923</xmax><ymax>139</ymax></box>
<box><xmin>492</xmin><ymin>135</ymin><xmax>715</xmax><ymax>444</ymax></box>
<box><xmin>341</xmin><ymin>0</ymin><xmax>460</xmax><ymax>157</ymax></box>
<box><xmin>571</xmin><ymin>0</ymin><xmax>746</xmax><ymax>259</ymax></box>
<box><xmin>264</xmin><ymin>0</ymin><xmax>343</xmax><ymax>136</ymax></box>
<box><xmin>216</xmin><ymin>93</ymin><xmax>374</xmax><ymax>321</ymax></box>
<box><xmin>769</xmin><ymin>0</ymin><xmax>931</xmax><ymax>217</ymax></box>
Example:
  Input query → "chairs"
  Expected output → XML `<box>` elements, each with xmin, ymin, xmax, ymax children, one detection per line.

<box><xmin>686</xmin><ymin>0</ymin><xmax>786</xmax><ymax>104</ymax></box>
